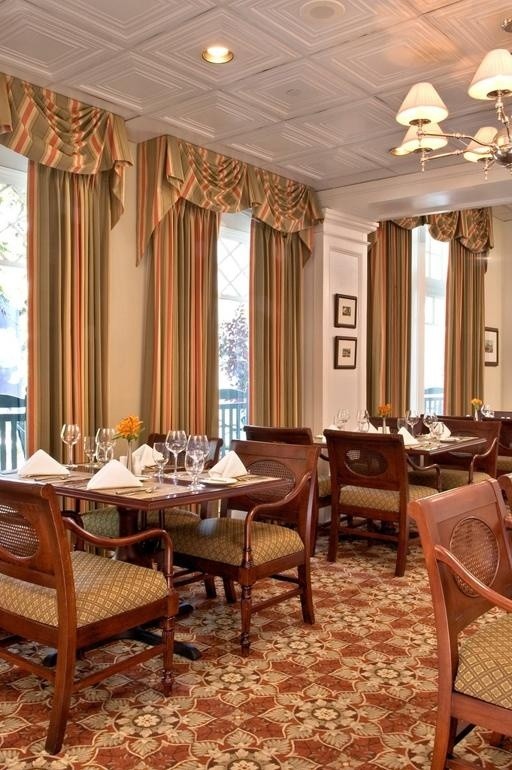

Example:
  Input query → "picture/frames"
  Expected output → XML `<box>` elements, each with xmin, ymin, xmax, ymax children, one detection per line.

<box><xmin>335</xmin><ymin>336</ymin><xmax>358</xmax><ymax>369</ymax></box>
<box><xmin>335</xmin><ymin>293</ymin><xmax>358</xmax><ymax>328</ymax></box>
<box><xmin>484</xmin><ymin>327</ymin><xmax>499</xmax><ymax>366</ymax></box>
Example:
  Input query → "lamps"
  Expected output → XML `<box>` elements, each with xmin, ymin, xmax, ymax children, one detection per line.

<box><xmin>396</xmin><ymin>20</ymin><xmax>512</xmax><ymax>180</ymax></box>
<box><xmin>201</xmin><ymin>46</ymin><xmax>235</xmax><ymax>64</ymax></box>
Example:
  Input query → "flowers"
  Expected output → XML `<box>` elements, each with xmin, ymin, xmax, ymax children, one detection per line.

<box><xmin>377</xmin><ymin>403</ymin><xmax>392</xmax><ymax>420</ymax></box>
<box><xmin>112</xmin><ymin>416</ymin><xmax>146</xmax><ymax>441</ymax></box>
<box><xmin>471</xmin><ymin>398</ymin><xmax>483</xmax><ymax>410</ymax></box>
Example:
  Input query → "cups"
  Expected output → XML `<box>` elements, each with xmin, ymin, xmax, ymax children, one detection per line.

<box><xmin>406</xmin><ymin>410</ymin><xmax>419</xmax><ymax>438</ymax></box>
<box><xmin>166</xmin><ymin>431</ymin><xmax>185</xmax><ymax>478</ymax></box>
<box><xmin>59</xmin><ymin>424</ymin><xmax>79</xmax><ymax>468</ymax></box>
<box><xmin>182</xmin><ymin>451</ymin><xmax>205</xmax><ymax>487</ymax></box>
<box><xmin>96</xmin><ymin>443</ymin><xmax>111</xmax><ymax>466</ymax></box>
<box><xmin>95</xmin><ymin>429</ymin><xmax>114</xmax><ymax>464</ymax></box>
<box><xmin>397</xmin><ymin>418</ymin><xmax>408</xmax><ymax>433</ymax></box>
<box><xmin>423</xmin><ymin>412</ymin><xmax>437</xmax><ymax>441</ymax></box>
<box><xmin>431</xmin><ymin>422</ymin><xmax>443</xmax><ymax>445</ymax></box>
<box><xmin>83</xmin><ymin>437</ymin><xmax>96</xmax><ymax>470</ymax></box>
<box><xmin>184</xmin><ymin>435</ymin><xmax>206</xmax><ymax>490</ymax></box>
<box><xmin>152</xmin><ymin>442</ymin><xmax>170</xmax><ymax>487</ymax></box>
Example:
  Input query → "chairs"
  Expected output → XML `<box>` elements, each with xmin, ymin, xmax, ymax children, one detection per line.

<box><xmin>323</xmin><ymin>428</ymin><xmax>440</xmax><ymax>577</ymax></box>
<box><xmin>243</xmin><ymin>424</ymin><xmax>354</xmax><ymax>560</ymax></box>
<box><xmin>408</xmin><ymin>420</ymin><xmax>502</xmax><ymax>491</ymax></box>
<box><xmin>159</xmin><ymin>441</ymin><xmax>323</xmax><ymax>660</ymax></box>
<box><xmin>408</xmin><ymin>477</ymin><xmax>512</xmax><ymax>770</ymax></box>
<box><xmin>2</xmin><ymin>479</ymin><xmax>179</xmax><ymax>755</ymax></box>
<box><xmin>70</xmin><ymin>434</ymin><xmax>224</xmax><ymax>599</ymax></box>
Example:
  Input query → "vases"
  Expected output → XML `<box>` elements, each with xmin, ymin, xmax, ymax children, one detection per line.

<box><xmin>126</xmin><ymin>442</ymin><xmax>134</xmax><ymax>475</ymax></box>
<box><xmin>382</xmin><ymin>417</ymin><xmax>386</xmax><ymax>434</ymax></box>
<box><xmin>474</xmin><ymin>409</ymin><xmax>478</xmax><ymax>420</ymax></box>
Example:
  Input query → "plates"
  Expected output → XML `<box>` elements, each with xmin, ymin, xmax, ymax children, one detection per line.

<box><xmin>134</xmin><ymin>475</ymin><xmax>151</xmax><ymax>480</ymax></box>
<box><xmin>198</xmin><ymin>477</ymin><xmax>237</xmax><ymax>485</ymax></box>
